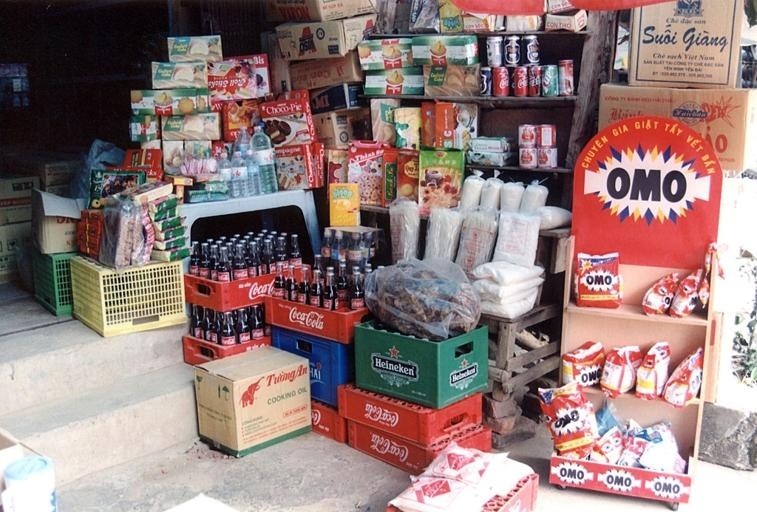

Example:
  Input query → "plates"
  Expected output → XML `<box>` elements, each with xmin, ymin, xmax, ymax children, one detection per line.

<box><xmin>262</xmin><ymin>118</ymin><xmax>296</xmax><ymax>148</ymax></box>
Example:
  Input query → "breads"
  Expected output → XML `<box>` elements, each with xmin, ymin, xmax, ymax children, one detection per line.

<box><xmin>189</xmin><ymin>41</ymin><xmax>209</xmax><ymax>55</ymax></box>
<box><xmin>173</xmin><ymin>67</ymin><xmax>194</xmax><ymax>82</ymax></box>
<box><xmin>264</xmin><ymin>120</ymin><xmax>291</xmax><ymax>144</ymax></box>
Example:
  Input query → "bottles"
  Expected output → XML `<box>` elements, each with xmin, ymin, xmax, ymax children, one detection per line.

<box><xmin>188</xmin><ymin>225</ymin><xmax>383</xmax><ymax>359</ymax></box>
<box><xmin>218</xmin><ymin>126</ymin><xmax>279</xmax><ymax>198</ymax></box>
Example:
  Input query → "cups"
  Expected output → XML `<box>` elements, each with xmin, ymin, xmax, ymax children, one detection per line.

<box><xmin>385</xmin><ymin>78</ymin><xmax>402</xmax><ymax>95</ymax></box>
<box><xmin>430</xmin><ymin>50</ymin><xmax>448</xmax><ymax>66</ymax></box>
<box><xmin>384</xmin><ymin>57</ymin><xmax>403</xmax><ymax>68</ymax></box>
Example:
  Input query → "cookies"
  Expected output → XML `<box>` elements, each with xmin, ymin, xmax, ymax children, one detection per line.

<box><xmin>198</xmin><ymin>97</ymin><xmax>206</xmax><ymax>112</ymax></box>
<box><xmin>131</xmin><ymin>90</ymin><xmax>143</xmax><ymax>102</ymax></box>
<box><xmin>178</xmin><ymin>98</ymin><xmax>195</xmax><ymax>113</ymax></box>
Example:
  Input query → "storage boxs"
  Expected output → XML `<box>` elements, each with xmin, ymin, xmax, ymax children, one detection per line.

<box><xmin>628</xmin><ymin>0</ymin><xmax>742</xmax><ymax>89</ymax></box>
<box><xmin>35</xmin><ymin>160</ymin><xmax>82</xmax><ymax>186</ymax></box>
<box><xmin>274</xmin><ymin>14</ymin><xmax>379</xmax><ymax>61</ymax></box>
<box><xmin>43</xmin><ymin>184</ymin><xmax>69</xmax><ymax>195</ymax></box>
<box><xmin>194</xmin><ymin>346</ymin><xmax>311</xmax><ymax>458</ymax></box>
<box><xmin>311</xmin><ymin>83</ymin><xmax>363</xmax><ymax>112</ymax></box>
<box><xmin>106</xmin><ymin>35</ymin><xmax>324</xmax><ymax>189</ymax></box>
<box><xmin>598</xmin><ymin>82</ymin><xmax>756</xmax><ymax>176</ymax></box>
<box><xmin>272</xmin><ymin>0</ymin><xmax>378</xmax><ymax>22</ymax></box>
<box><xmin>309</xmin><ymin>106</ymin><xmax>370</xmax><ymax>151</ymax></box>
<box><xmin>354</xmin><ymin>320</ymin><xmax>491</xmax><ymax>408</ymax></box>
<box><xmin>0</xmin><ymin>173</ymin><xmax>40</xmax><ymax>225</ymax></box>
<box><xmin>289</xmin><ymin>50</ymin><xmax>365</xmax><ymax>91</ymax></box>
<box><xmin>0</xmin><ymin>222</ymin><xmax>31</xmax><ymax>286</ymax></box>
<box><xmin>30</xmin><ymin>187</ymin><xmax>85</xmax><ymax>255</ymax></box>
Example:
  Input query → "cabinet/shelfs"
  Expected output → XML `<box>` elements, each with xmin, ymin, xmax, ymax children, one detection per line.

<box><xmin>549</xmin><ymin>231</ymin><xmax>720</xmax><ymax>512</ymax></box>
<box><xmin>360</xmin><ymin>30</ymin><xmax>605</xmax><ymax>325</ymax></box>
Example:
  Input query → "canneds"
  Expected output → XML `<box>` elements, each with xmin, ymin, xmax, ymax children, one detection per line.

<box><xmin>519</xmin><ymin>146</ymin><xmax>538</xmax><ymax>169</ymax></box>
<box><xmin>537</xmin><ymin>148</ymin><xmax>558</xmax><ymax>170</ymax></box>
<box><xmin>537</xmin><ymin>124</ymin><xmax>557</xmax><ymax>149</ymax></box>
<box><xmin>503</xmin><ymin>36</ymin><xmax>522</xmax><ymax>67</ymax></box>
<box><xmin>527</xmin><ymin>65</ymin><xmax>542</xmax><ymax>97</ymax></box>
<box><xmin>558</xmin><ymin>59</ymin><xmax>574</xmax><ymax>94</ymax></box>
<box><xmin>479</xmin><ymin>67</ymin><xmax>492</xmax><ymax>96</ymax></box>
<box><xmin>542</xmin><ymin>65</ymin><xmax>559</xmax><ymax>97</ymax></box>
<box><xmin>492</xmin><ymin>67</ymin><xmax>510</xmax><ymax>97</ymax></box>
<box><xmin>514</xmin><ymin>66</ymin><xmax>527</xmax><ymax>96</ymax></box>
<box><xmin>520</xmin><ymin>35</ymin><xmax>541</xmax><ymax>65</ymax></box>
<box><xmin>518</xmin><ymin>124</ymin><xmax>537</xmax><ymax>148</ymax></box>
<box><xmin>486</xmin><ymin>36</ymin><xmax>503</xmax><ymax>68</ymax></box>
